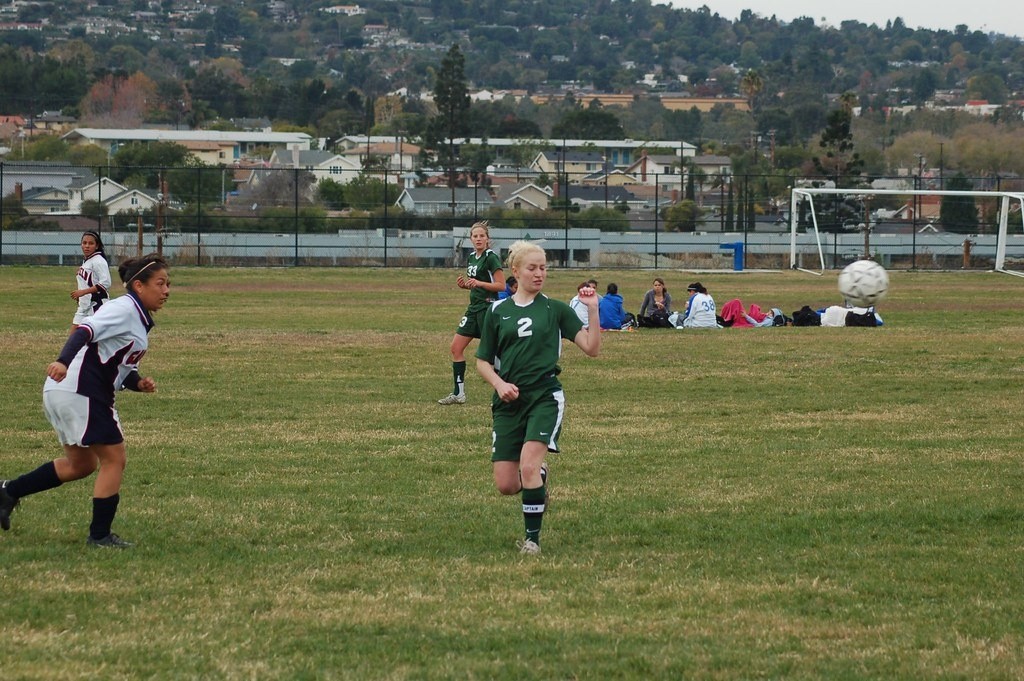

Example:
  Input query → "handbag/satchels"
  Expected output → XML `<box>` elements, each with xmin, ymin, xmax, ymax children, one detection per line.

<box><xmin>774</xmin><ymin>315</ymin><xmax>792</xmax><ymax>326</ymax></box>
<box><xmin>653</xmin><ymin>311</ymin><xmax>674</xmax><ymax>328</ymax></box>
<box><xmin>793</xmin><ymin>306</ymin><xmax>821</xmax><ymax>326</ymax></box>
<box><xmin>623</xmin><ymin>313</ymin><xmax>637</xmax><ymax>327</ymax></box>
<box><xmin>637</xmin><ymin>314</ymin><xmax>653</xmax><ymax>327</ymax></box>
<box><xmin>845</xmin><ymin>311</ymin><xmax>877</xmax><ymax>327</ymax></box>
<box><xmin>716</xmin><ymin>315</ymin><xmax>734</xmax><ymax>327</ymax></box>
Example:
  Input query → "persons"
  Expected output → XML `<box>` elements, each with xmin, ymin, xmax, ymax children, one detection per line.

<box><xmin>476</xmin><ymin>239</ymin><xmax>602</xmax><ymax>556</ymax></box>
<box><xmin>637</xmin><ymin>278</ymin><xmax>674</xmax><ymax>328</ymax></box>
<box><xmin>570</xmin><ymin>282</ymin><xmax>589</xmax><ymax>330</ymax></box>
<box><xmin>438</xmin><ymin>218</ymin><xmax>506</xmax><ymax>405</ymax></box>
<box><xmin>675</xmin><ymin>282</ymin><xmax>717</xmax><ymax>330</ymax></box>
<box><xmin>68</xmin><ymin>231</ymin><xmax>112</xmax><ymax>337</ymax></box>
<box><xmin>0</xmin><ymin>252</ymin><xmax>172</xmax><ymax>550</ymax></box>
<box><xmin>497</xmin><ymin>276</ymin><xmax>518</xmax><ymax>300</ymax></box>
<box><xmin>598</xmin><ymin>284</ymin><xmax>638</xmax><ymax>329</ymax></box>
<box><xmin>587</xmin><ymin>279</ymin><xmax>603</xmax><ymax>300</ymax></box>
<box><xmin>716</xmin><ymin>300</ymin><xmax>782</xmax><ymax>329</ymax></box>
<box><xmin>789</xmin><ymin>305</ymin><xmax>876</xmax><ymax>328</ymax></box>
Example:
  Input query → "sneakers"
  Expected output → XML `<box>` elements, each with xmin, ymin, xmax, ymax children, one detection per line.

<box><xmin>438</xmin><ymin>393</ymin><xmax>466</xmax><ymax>405</ymax></box>
<box><xmin>0</xmin><ymin>480</ymin><xmax>21</xmax><ymax>530</ymax></box>
<box><xmin>88</xmin><ymin>533</ymin><xmax>133</xmax><ymax>550</ymax></box>
<box><xmin>515</xmin><ymin>537</ymin><xmax>541</xmax><ymax>555</ymax></box>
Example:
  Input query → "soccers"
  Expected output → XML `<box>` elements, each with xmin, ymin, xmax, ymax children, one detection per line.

<box><xmin>836</xmin><ymin>259</ymin><xmax>891</xmax><ymax>309</ymax></box>
